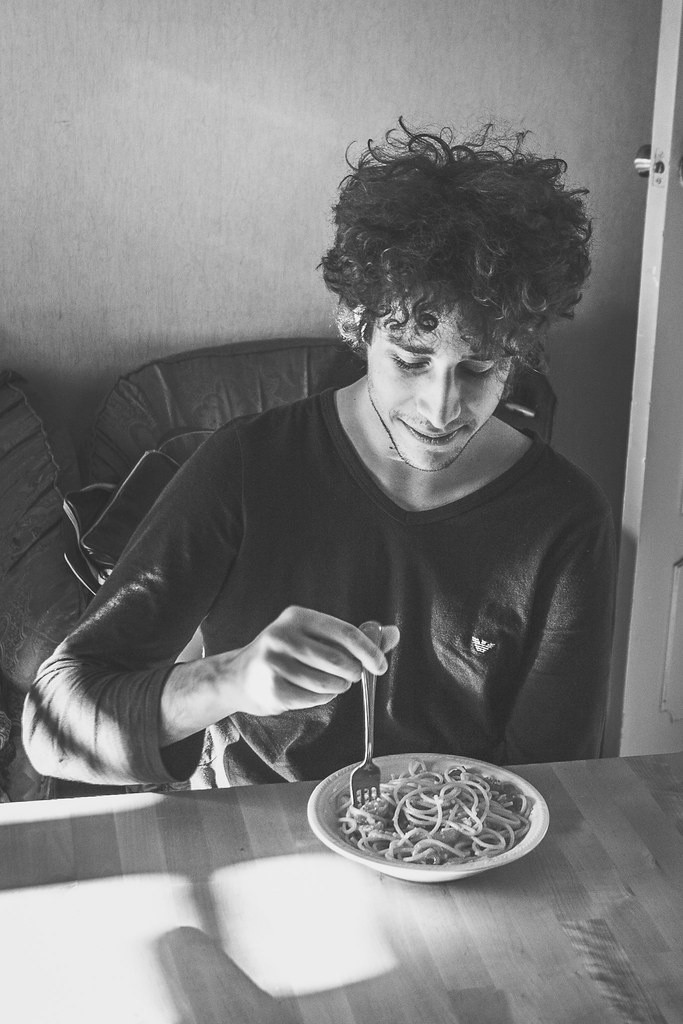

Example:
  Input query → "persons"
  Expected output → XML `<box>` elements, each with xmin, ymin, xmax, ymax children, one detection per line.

<box><xmin>20</xmin><ymin>138</ymin><xmax>617</xmax><ymax>798</ymax></box>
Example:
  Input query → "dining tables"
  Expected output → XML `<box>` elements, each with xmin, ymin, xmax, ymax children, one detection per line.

<box><xmin>0</xmin><ymin>749</ymin><xmax>683</xmax><ymax>1024</ymax></box>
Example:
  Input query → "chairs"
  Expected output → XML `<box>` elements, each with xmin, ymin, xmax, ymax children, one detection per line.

<box><xmin>54</xmin><ymin>336</ymin><xmax>557</xmax><ymax>596</ymax></box>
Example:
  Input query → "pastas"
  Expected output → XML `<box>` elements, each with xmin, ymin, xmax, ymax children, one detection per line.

<box><xmin>333</xmin><ymin>754</ymin><xmax>533</xmax><ymax>864</ymax></box>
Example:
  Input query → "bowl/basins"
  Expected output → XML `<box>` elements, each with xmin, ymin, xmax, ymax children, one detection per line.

<box><xmin>308</xmin><ymin>752</ymin><xmax>550</xmax><ymax>882</ymax></box>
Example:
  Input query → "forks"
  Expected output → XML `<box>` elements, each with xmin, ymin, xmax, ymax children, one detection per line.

<box><xmin>350</xmin><ymin>621</ymin><xmax>382</xmax><ymax>808</ymax></box>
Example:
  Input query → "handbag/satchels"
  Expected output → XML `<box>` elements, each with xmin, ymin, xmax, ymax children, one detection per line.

<box><xmin>63</xmin><ymin>449</ymin><xmax>181</xmax><ymax>597</ymax></box>
